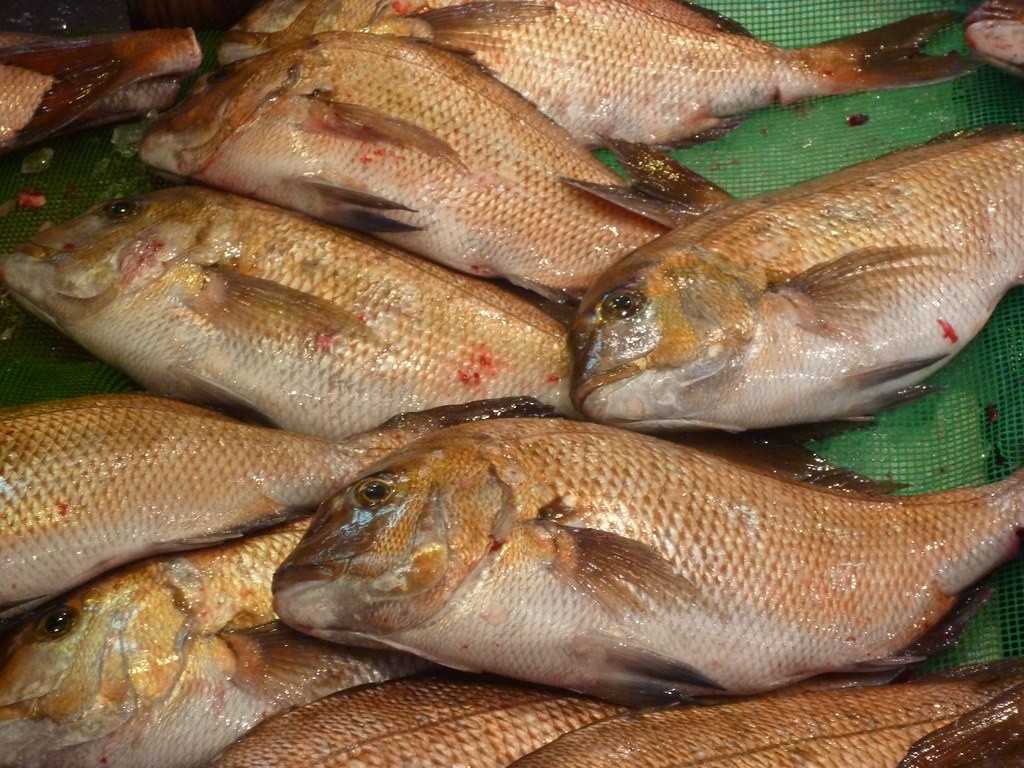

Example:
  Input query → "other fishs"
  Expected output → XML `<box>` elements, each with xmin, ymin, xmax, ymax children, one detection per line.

<box><xmin>1</xmin><ymin>0</ymin><xmax>1024</xmax><ymax>768</ymax></box>
